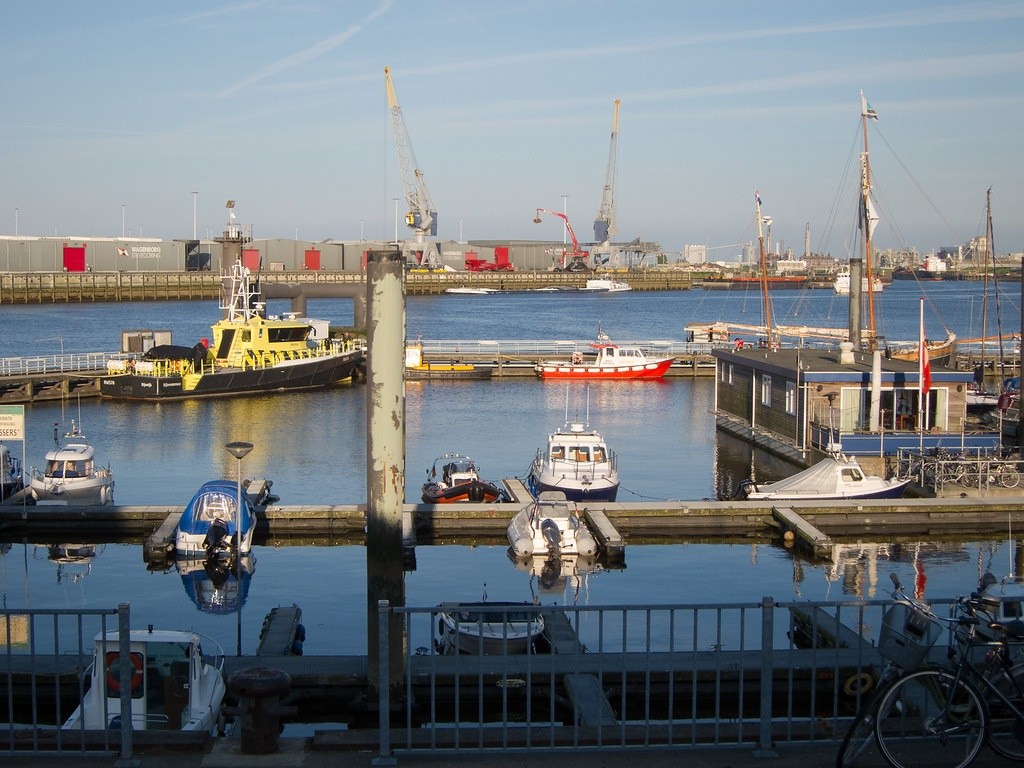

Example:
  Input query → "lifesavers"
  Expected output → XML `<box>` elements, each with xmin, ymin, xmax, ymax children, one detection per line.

<box><xmin>843</xmin><ymin>673</ymin><xmax>874</xmax><ymax>697</ymax></box>
<box><xmin>106</xmin><ymin>651</ymin><xmax>142</xmax><ymax>691</ymax></box>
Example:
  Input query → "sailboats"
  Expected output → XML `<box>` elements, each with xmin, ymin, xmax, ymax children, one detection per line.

<box><xmin>778</xmin><ymin>86</ymin><xmax>1020</xmax><ymax>438</ymax></box>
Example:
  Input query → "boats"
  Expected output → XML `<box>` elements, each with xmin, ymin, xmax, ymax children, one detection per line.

<box><xmin>420</xmin><ymin>451</ymin><xmax>501</xmax><ymax>503</ymax></box>
<box><xmin>438</xmin><ymin>582</ymin><xmax>545</xmax><ymax>654</ymax></box>
<box><xmin>526</xmin><ymin>384</ymin><xmax>621</xmax><ymax>503</ymax></box>
<box><xmin>891</xmin><ymin>266</ymin><xmax>960</xmax><ymax>281</ymax></box>
<box><xmin>403</xmin><ymin>364</ymin><xmax>494</xmax><ymax>381</ymax></box>
<box><xmin>506</xmin><ymin>490</ymin><xmax>599</xmax><ymax>558</ymax></box>
<box><xmin>532</xmin><ymin>320</ymin><xmax>676</xmax><ymax>380</ymax></box>
<box><xmin>29</xmin><ymin>389</ymin><xmax>117</xmax><ymax>507</ymax></box>
<box><xmin>61</xmin><ymin>625</ymin><xmax>227</xmax><ymax>739</ymax></box>
<box><xmin>832</xmin><ymin>272</ymin><xmax>883</xmax><ymax>293</ymax></box>
<box><xmin>0</xmin><ymin>444</ymin><xmax>23</xmax><ymax>501</ymax></box>
<box><xmin>730</xmin><ymin>406</ymin><xmax>913</xmax><ymax>503</ymax></box>
<box><xmin>175</xmin><ymin>478</ymin><xmax>257</xmax><ymax>557</ymax></box>
<box><xmin>99</xmin><ymin>254</ymin><xmax>364</xmax><ymax>401</ymax></box>
<box><xmin>585</xmin><ymin>279</ymin><xmax>633</xmax><ymax>292</ymax></box>
<box><xmin>701</xmin><ymin>275</ymin><xmax>815</xmax><ymax>289</ymax></box>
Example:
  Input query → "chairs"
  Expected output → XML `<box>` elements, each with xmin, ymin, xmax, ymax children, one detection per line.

<box><xmin>170</xmin><ymin>659</ymin><xmax>190</xmax><ymax>688</ymax></box>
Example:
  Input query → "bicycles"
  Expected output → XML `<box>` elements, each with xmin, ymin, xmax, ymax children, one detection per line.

<box><xmin>881</xmin><ymin>447</ymin><xmax>1021</xmax><ymax>489</ymax></box>
<box><xmin>833</xmin><ymin>573</ymin><xmax>1024</xmax><ymax>768</ymax></box>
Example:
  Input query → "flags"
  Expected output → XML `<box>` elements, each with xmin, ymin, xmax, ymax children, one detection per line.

<box><xmin>917</xmin><ymin>328</ymin><xmax>932</xmax><ymax>394</ymax></box>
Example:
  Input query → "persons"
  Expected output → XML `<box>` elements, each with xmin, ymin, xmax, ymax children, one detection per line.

<box><xmin>734</xmin><ymin>338</ymin><xmax>744</xmax><ymax>349</ymax></box>
<box><xmin>708</xmin><ymin>328</ymin><xmax>713</xmax><ymax>342</ymax></box>
<box><xmin>344</xmin><ymin>331</ymin><xmax>351</xmax><ymax>343</ymax></box>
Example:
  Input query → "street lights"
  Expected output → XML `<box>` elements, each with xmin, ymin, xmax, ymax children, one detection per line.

<box><xmin>224</xmin><ymin>442</ymin><xmax>254</xmax><ymax>582</ymax></box>
<box><xmin>15</xmin><ymin>207</ymin><xmax>18</xmax><ymax>237</ymax></box>
<box><xmin>191</xmin><ymin>191</ymin><xmax>199</xmax><ymax>240</ymax></box>
<box><xmin>121</xmin><ymin>204</ymin><xmax>126</xmax><ymax>237</ymax></box>
<box><xmin>359</xmin><ymin>218</ymin><xmax>364</xmax><ymax>241</ymax></box>
<box><xmin>392</xmin><ymin>197</ymin><xmax>399</xmax><ymax>244</ymax></box>
<box><xmin>560</xmin><ymin>195</ymin><xmax>568</xmax><ymax>269</ymax></box>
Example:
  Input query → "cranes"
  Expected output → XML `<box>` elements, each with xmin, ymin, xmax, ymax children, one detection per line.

<box><xmin>382</xmin><ymin>64</ymin><xmax>442</xmax><ymax>270</ymax></box>
<box><xmin>587</xmin><ymin>95</ymin><xmax>623</xmax><ymax>270</ymax></box>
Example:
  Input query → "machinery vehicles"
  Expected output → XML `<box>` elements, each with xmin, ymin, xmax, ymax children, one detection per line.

<box><xmin>532</xmin><ymin>208</ymin><xmax>589</xmax><ymax>272</ymax></box>
